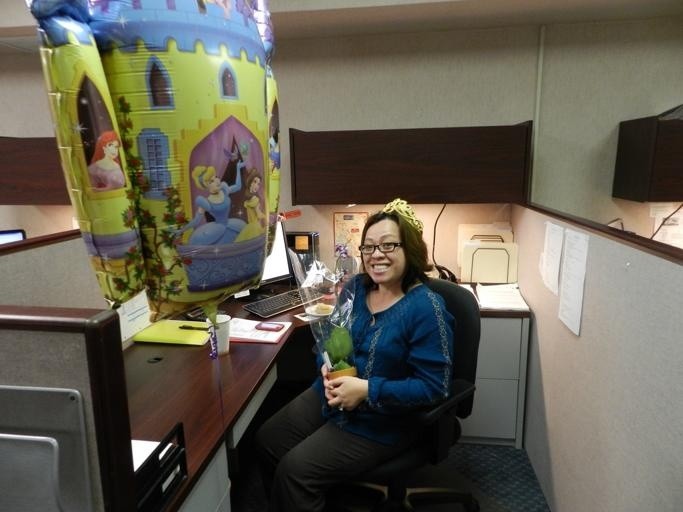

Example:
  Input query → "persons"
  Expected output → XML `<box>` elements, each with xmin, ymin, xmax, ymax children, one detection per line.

<box><xmin>167</xmin><ymin>159</ymin><xmax>267</xmax><ymax>246</ymax></box>
<box><xmin>87</xmin><ymin>131</ymin><xmax>125</xmax><ymax>191</ymax></box>
<box><xmin>239</xmin><ymin>210</ymin><xmax>455</xmax><ymax>512</ymax></box>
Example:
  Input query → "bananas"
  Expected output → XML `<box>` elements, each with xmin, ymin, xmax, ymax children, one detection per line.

<box><xmin>241</xmin><ymin>287</ymin><xmax>324</xmax><ymax>318</ymax></box>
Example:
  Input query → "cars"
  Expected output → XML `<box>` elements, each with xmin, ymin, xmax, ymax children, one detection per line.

<box><xmin>205</xmin><ymin>314</ymin><xmax>232</xmax><ymax>355</ymax></box>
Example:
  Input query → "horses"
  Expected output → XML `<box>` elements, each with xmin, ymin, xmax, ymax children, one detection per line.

<box><xmin>256</xmin><ymin>322</ymin><xmax>284</xmax><ymax>331</ymax></box>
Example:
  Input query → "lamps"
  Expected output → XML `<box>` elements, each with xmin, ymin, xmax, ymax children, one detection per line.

<box><xmin>330</xmin><ymin>271</ymin><xmax>480</xmax><ymax>511</ymax></box>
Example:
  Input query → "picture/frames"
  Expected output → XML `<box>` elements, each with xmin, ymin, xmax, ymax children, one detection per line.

<box><xmin>120</xmin><ymin>270</ymin><xmax>530</xmax><ymax>510</ymax></box>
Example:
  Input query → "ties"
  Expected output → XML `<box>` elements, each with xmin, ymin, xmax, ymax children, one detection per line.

<box><xmin>0</xmin><ymin>229</ymin><xmax>26</xmax><ymax>245</ymax></box>
<box><xmin>243</xmin><ymin>219</ymin><xmax>295</xmax><ymax>303</ymax></box>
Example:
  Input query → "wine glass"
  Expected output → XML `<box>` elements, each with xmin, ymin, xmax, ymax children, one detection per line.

<box><xmin>338</xmin><ymin>405</ymin><xmax>344</xmax><ymax>412</ymax></box>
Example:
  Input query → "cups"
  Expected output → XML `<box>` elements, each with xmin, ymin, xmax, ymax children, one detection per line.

<box><xmin>358</xmin><ymin>242</ymin><xmax>403</xmax><ymax>255</ymax></box>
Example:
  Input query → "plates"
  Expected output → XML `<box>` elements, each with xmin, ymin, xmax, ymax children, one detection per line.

<box><xmin>287</xmin><ymin>121</ymin><xmax>532</xmax><ymax>206</ymax></box>
<box><xmin>613</xmin><ymin>116</ymin><xmax>682</xmax><ymax>202</ymax></box>
